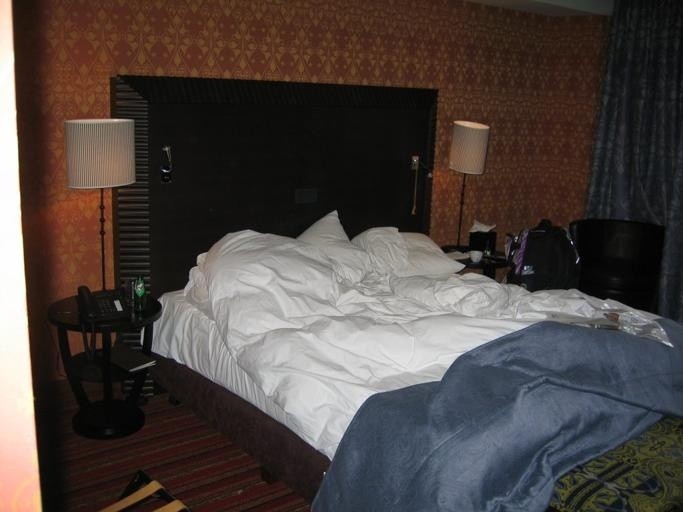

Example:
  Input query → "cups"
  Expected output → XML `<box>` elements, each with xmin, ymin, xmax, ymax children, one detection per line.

<box><xmin>469</xmin><ymin>250</ymin><xmax>483</xmax><ymax>263</ymax></box>
<box><xmin>125</xmin><ymin>279</ymin><xmax>135</xmax><ymax>305</ymax></box>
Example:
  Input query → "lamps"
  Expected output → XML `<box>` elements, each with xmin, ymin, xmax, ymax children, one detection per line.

<box><xmin>448</xmin><ymin>120</ymin><xmax>490</xmax><ymax>250</ymax></box>
<box><xmin>65</xmin><ymin>118</ymin><xmax>137</xmax><ymax>291</ymax></box>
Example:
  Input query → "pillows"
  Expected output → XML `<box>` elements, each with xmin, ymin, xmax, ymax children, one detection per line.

<box><xmin>296</xmin><ymin>210</ymin><xmax>374</xmax><ymax>284</ymax></box>
<box><xmin>393</xmin><ymin>232</ymin><xmax>466</xmax><ymax>277</ymax></box>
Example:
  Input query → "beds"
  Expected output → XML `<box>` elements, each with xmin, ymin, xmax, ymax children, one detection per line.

<box><xmin>110</xmin><ymin>75</ymin><xmax>683</xmax><ymax>512</ymax></box>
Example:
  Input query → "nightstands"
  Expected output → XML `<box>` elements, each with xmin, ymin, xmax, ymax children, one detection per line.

<box><xmin>440</xmin><ymin>246</ymin><xmax>508</xmax><ymax>279</ymax></box>
<box><xmin>47</xmin><ymin>290</ymin><xmax>163</xmax><ymax>440</ymax></box>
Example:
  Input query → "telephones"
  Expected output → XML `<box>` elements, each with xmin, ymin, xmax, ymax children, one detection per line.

<box><xmin>78</xmin><ymin>285</ymin><xmax>130</xmax><ymax>322</ymax></box>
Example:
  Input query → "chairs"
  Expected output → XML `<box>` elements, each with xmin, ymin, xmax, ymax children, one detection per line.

<box><xmin>562</xmin><ymin>218</ymin><xmax>666</xmax><ymax>314</ymax></box>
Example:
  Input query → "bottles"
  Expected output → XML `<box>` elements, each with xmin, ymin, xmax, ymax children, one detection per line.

<box><xmin>134</xmin><ymin>275</ymin><xmax>146</xmax><ymax>312</ymax></box>
